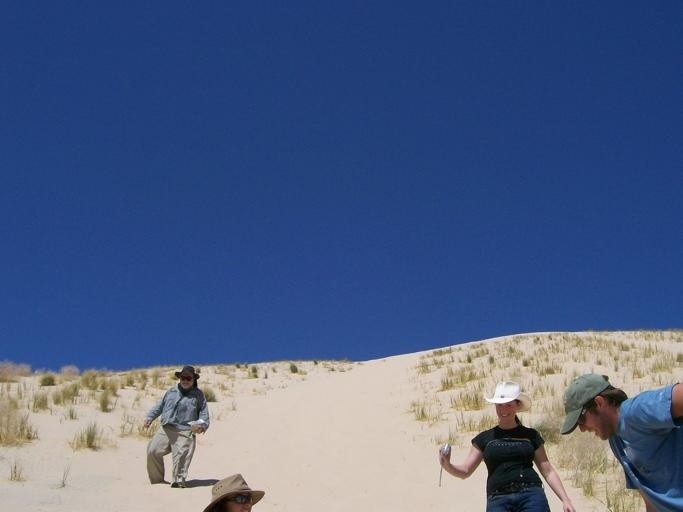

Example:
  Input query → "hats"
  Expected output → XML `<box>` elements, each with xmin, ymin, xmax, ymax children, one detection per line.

<box><xmin>560</xmin><ymin>374</ymin><xmax>610</xmax><ymax>434</ymax></box>
<box><xmin>175</xmin><ymin>365</ymin><xmax>200</xmax><ymax>379</ymax></box>
<box><xmin>484</xmin><ymin>380</ymin><xmax>532</xmax><ymax>413</ymax></box>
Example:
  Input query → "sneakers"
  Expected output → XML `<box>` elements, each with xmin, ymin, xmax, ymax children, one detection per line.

<box><xmin>170</xmin><ymin>482</ymin><xmax>183</xmax><ymax>488</ymax></box>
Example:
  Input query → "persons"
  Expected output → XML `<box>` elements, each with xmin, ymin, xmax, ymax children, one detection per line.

<box><xmin>143</xmin><ymin>366</ymin><xmax>210</xmax><ymax>488</ymax></box>
<box><xmin>439</xmin><ymin>381</ymin><xmax>576</xmax><ymax>512</ymax></box>
<box><xmin>560</xmin><ymin>374</ymin><xmax>683</xmax><ymax>512</ymax></box>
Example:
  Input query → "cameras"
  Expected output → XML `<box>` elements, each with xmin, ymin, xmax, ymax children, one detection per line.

<box><xmin>440</xmin><ymin>442</ymin><xmax>451</xmax><ymax>456</ymax></box>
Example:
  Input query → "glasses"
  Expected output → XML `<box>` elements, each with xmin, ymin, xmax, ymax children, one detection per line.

<box><xmin>226</xmin><ymin>495</ymin><xmax>252</xmax><ymax>504</ymax></box>
<box><xmin>182</xmin><ymin>376</ymin><xmax>192</xmax><ymax>381</ymax></box>
<box><xmin>577</xmin><ymin>408</ymin><xmax>587</xmax><ymax>424</ymax></box>
